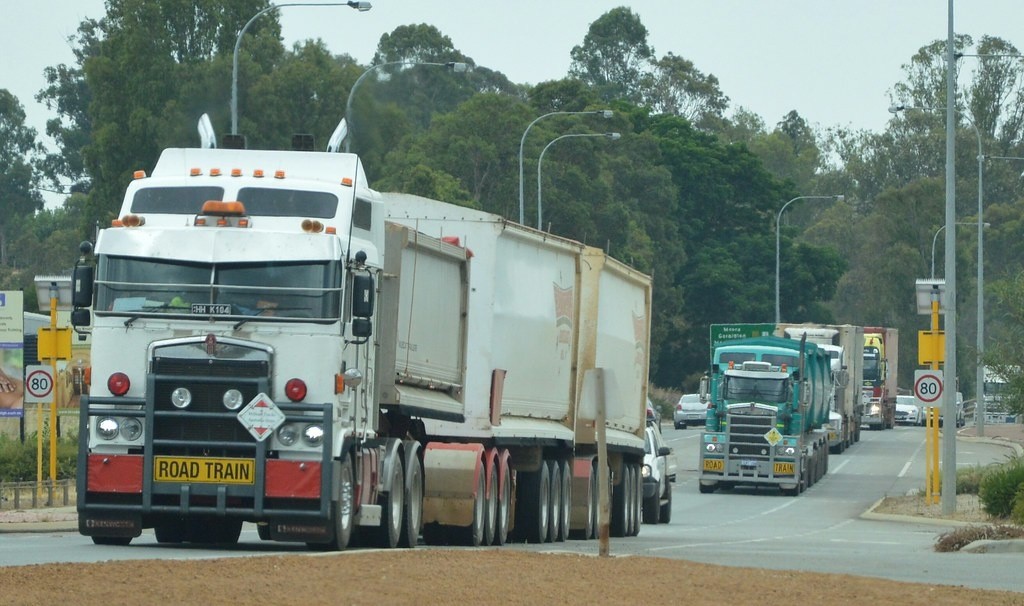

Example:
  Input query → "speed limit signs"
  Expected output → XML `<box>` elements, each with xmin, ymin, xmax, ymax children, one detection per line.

<box><xmin>915</xmin><ymin>374</ymin><xmax>943</xmax><ymax>403</ymax></box>
<box><xmin>27</xmin><ymin>369</ymin><xmax>54</xmax><ymax>398</ymax></box>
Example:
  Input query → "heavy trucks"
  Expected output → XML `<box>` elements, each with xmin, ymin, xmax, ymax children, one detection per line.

<box><xmin>698</xmin><ymin>331</ymin><xmax>833</xmax><ymax>497</ymax></box>
<box><xmin>774</xmin><ymin>322</ymin><xmax>864</xmax><ymax>453</ymax></box>
<box><xmin>71</xmin><ymin>114</ymin><xmax>655</xmax><ymax>553</ymax></box>
<box><xmin>862</xmin><ymin>326</ymin><xmax>898</xmax><ymax>430</ymax></box>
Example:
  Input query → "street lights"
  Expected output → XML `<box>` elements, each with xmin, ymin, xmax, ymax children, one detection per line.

<box><xmin>519</xmin><ymin>110</ymin><xmax>613</xmax><ymax>227</ymax></box>
<box><xmin>775</xmin><ymin>195</ymin><xmax>847</xmax><ymax>323</ymax></box>
<box><xmin>931</xmin><ymin>222</ymin><xmax>992</xmax><ymax>282</ymax></box>
<box><xmin>890</xmin><ymin>106</ymin><xmax>985</xmax><ymax>438</ymax></box>
<box><xmin>345</xmin><ymin>60</ymin><xmax>472</xmax><ymax>156</ymax></box>
<box><xmin>231</xmin><ymin>0</ymin><xmax>374</xmax><ymax>136</ymax></box>
<box><xmin>537</xmin><ymin>132</ymin><xmax>621</xmax><ymax>234</ymax></box>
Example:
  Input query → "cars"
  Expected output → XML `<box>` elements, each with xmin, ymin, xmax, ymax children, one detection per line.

<box><xmin>893</xmin><ymin>395</ymin><xmax>923</xmax><ymax>426</ymax></box>
<box><xmin>646</xmin><ymin>397</ymin><xmax>663</xmax><ymax>433</ymax></box>
<box><xmin>642</xmin><ymin>421</ymin><xmax>678</xmax><ymax>525</ymax></box>
<box><xmin>674</xmin><ymin>393</ymin><xmax>711</xmax><ymax>429</ymax></box>
<box><xmin>924</xmin><ymin>392</ymin><xmax>966</xmax><ymax>428</ymax></box>
